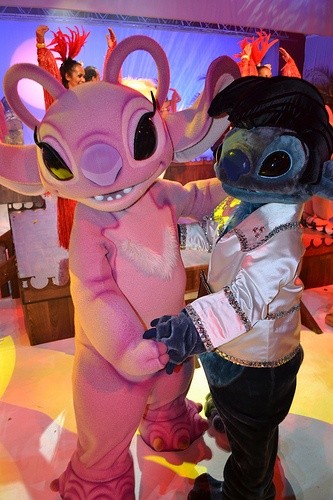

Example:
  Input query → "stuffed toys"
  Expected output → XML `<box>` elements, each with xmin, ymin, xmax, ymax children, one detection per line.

<box><xmin>139</xmin><ymin>74</ymin><xmax>333</xmax><ymax>500</ymax></box>
<box><xmin>0</xmin><ymin>33</ymin><xmax>237</xmax><ymax>500</ymax></box>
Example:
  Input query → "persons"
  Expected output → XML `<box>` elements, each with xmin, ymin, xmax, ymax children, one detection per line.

<box><xmin>35</xmin><ymin>24</ymin><xmax>86</xmax><ymax>253</ymax></box>
<box><xmin>238</xmin><ymin>42</ymin><xmax>302</xmax><ymax>77</ymax></box>
<box><xmin>82</xmin><ymin>27</ymin><xmax>123</xmax><ymax>83</ymax></box>
